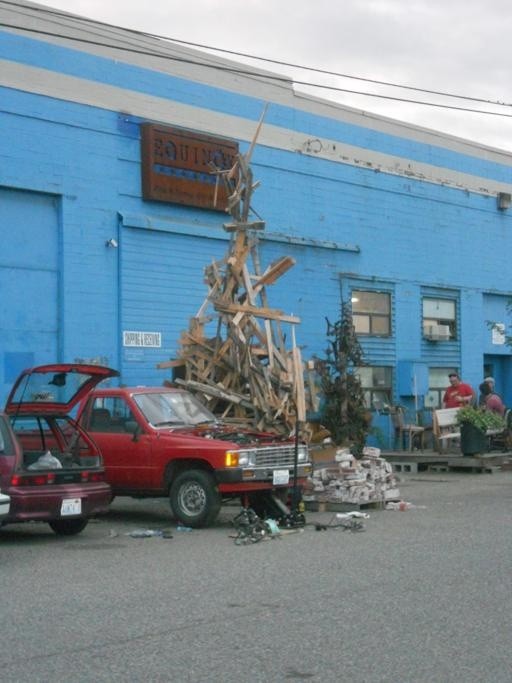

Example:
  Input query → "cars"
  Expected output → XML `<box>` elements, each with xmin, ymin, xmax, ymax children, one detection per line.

<box><xmin>0</xmin><ymin>363</ymin><xmax>121</xmax><ymax>535</ymax></box>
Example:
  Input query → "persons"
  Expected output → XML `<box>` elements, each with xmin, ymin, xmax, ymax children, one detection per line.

<box><xmin>442</xmin><ymin>373</ymin><xmax>476</xmax><ymax>408</ymax></box>
<box><xmin>479</xmin><ymin>382</ymin><xmax>505</xmax><ymax>419</ymax></box>
<box><xmin>479</xmin><ymin>377</ymin><xmax>503</xmax><ymax>407</ymax></box>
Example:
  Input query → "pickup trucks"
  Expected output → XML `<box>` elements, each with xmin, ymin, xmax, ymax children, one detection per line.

<box><xmin>14</xmin><ymin>386</ymin><xmax>314</xmax><ymax>528</ymax></box>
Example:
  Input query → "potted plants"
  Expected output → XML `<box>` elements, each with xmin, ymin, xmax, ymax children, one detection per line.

<box><xmin>453</xmin><ymin>403</ymin><xmax>507</xmax><ymax>455</ymax></box>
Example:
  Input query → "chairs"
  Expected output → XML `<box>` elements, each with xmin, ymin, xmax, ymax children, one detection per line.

<box><xmin>390</xmin><ymin>407</ymin><xmax>425</xmax><ymax>452</ymax></box>
<box><xmin>89</xmin><ymin>408</ymin><xmax>123</xmax><ymax>432</ymax></box>
